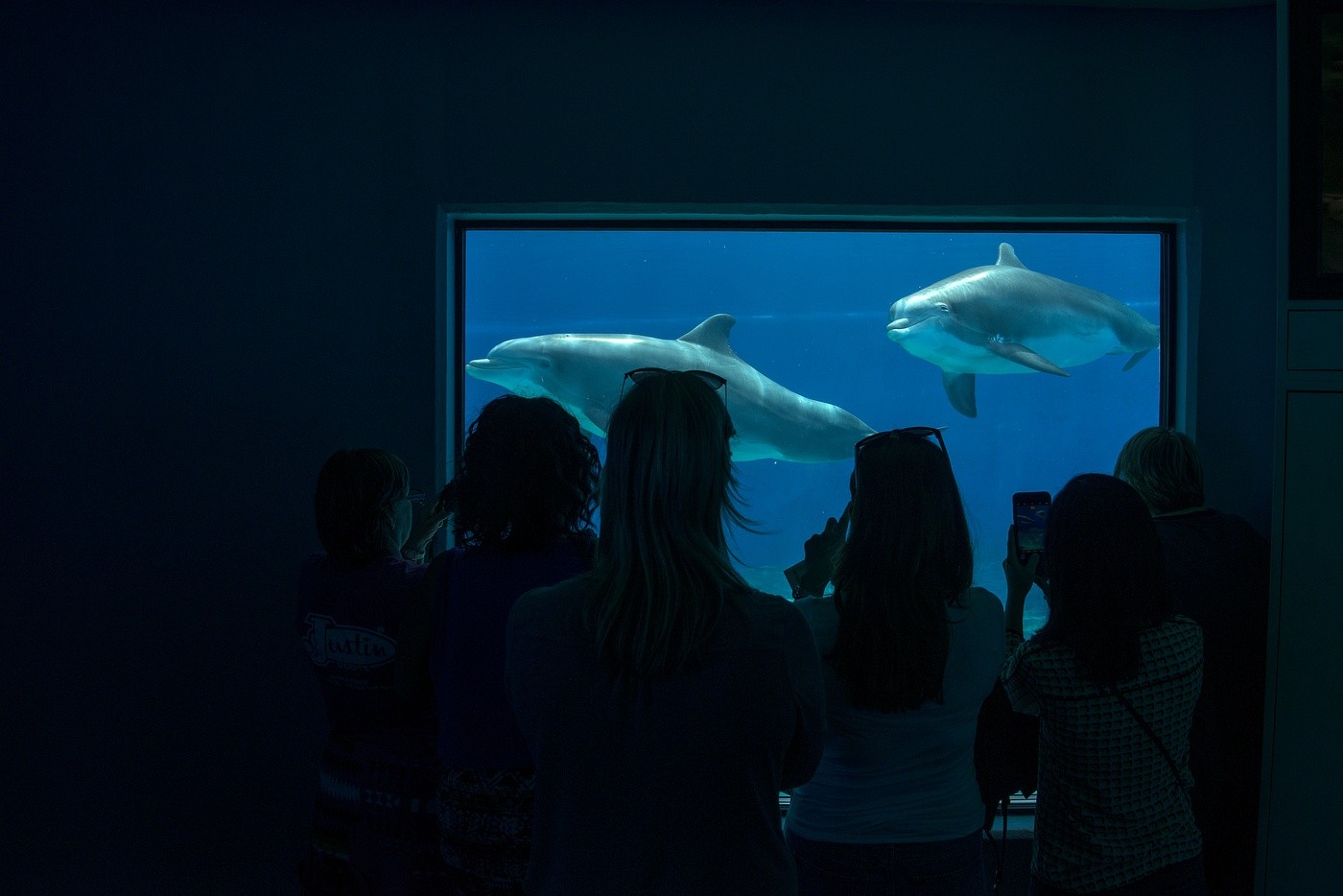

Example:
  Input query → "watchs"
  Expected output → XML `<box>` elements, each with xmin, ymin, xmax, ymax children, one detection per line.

<box><xmin>791</xmin><ymin>584</ymin><xmax>823</xmax><ymax>600</ymax></box>
<box><xmin>401</xmin><ymin>545</ymin><xmax>426</xmax><ymax>563</ymax></box>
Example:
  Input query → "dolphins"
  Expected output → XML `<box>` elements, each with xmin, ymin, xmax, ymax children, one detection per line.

<box><xmin>465</xmin><ymin>313</ymin><xmax>949</xmax><ymax>463</ymax></box>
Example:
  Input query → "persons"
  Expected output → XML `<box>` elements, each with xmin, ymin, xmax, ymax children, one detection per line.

<box><xmin>783</xmin><ymin>430</ymin><xmax>1005</xmax><ymax>896</ymax></box>
<box><xmin>1000</xmin><ymin>469</ymin><xmax>1212</xmax><ymax>896</ymax></box>
<box><xmin>291</xmin><ymin>447</ymin><xmax>449</xmax><ymax>895</ymax></box>
<box><xmin>1113</xmin><ymin>427</ymin><xmax>1269</xmax><ymax>896</ymax></box>
<box><xmin>420</xmin><ymin>395</ymin><xmax>613</xmax><ymax>896</ymax></box>
<box><xmin>497</xmin><ymin>367</ymin><xmax>834</xmax><ymax>896</ymax></box>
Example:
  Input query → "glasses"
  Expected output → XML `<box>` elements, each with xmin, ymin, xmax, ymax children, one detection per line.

<box><xmin>854</xmin><ymin>426</ymin><xmax>951</xmax><ymax>492</ymax></box>
<box><xmin>619</xmin><ymin>367</ymin><xmax>728</xmax><ymax>416</ymax></box>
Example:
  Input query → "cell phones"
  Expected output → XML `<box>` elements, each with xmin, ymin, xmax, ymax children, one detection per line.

<box><xmin>1012</xmin><ymin>490</ymin><xmax>1054</xmax><ymax>574</ymax></box>
